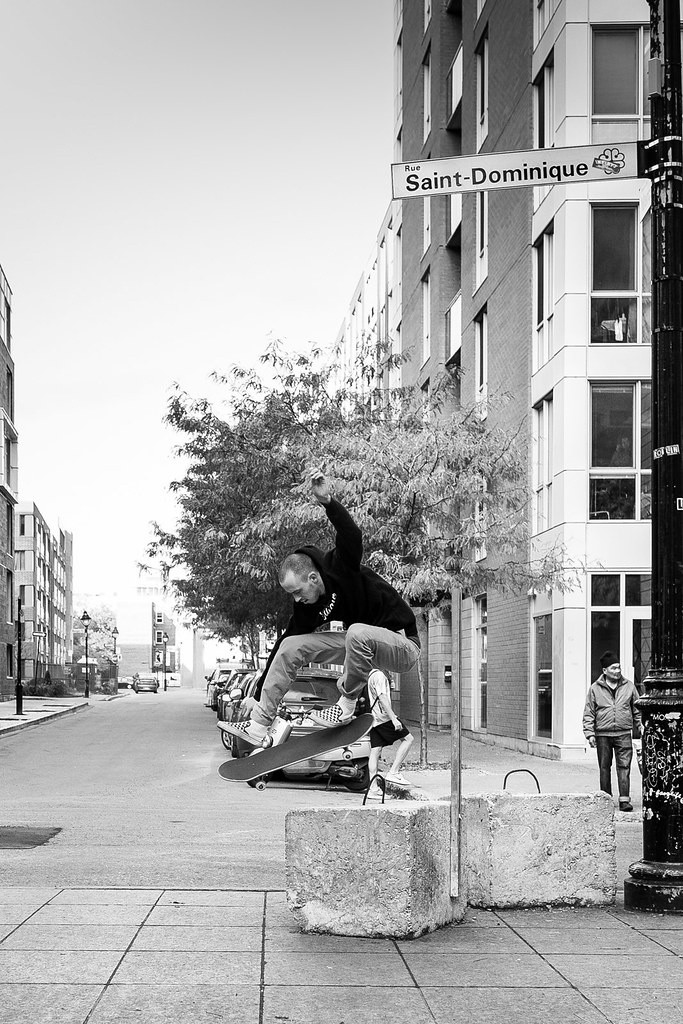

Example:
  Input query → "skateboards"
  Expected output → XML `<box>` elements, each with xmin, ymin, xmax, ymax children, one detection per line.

<box><xmin>216</xmin><ymin>712</ymin><xmax>376</xmax><ymax>790</ymax></box>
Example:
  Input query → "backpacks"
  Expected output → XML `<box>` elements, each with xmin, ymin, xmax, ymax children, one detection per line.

<box><xmin>352</xmin><ymin>686</ymin><xmax>371</xmax><ymax>716</ymax></box>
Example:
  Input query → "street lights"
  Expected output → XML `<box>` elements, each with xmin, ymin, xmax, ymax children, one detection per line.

<box><xmin>80</xmin><ymin>611</ymin><xmax>92</xmax><ymax>698</ymax></box>
<box><xmin>111</xmin><ymin>626</ymin><xmax>119</xmax><ymax>654</ymax></box>
<box><xmin>162</xmin><ymin>632</ymin><xmax>169</xmax><ymax>690</ymax></box>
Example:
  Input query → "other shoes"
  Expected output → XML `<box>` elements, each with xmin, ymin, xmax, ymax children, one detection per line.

<box><xmin>619</xmin><ymin>800</ymin><xmax>633</xmax><ymax>812</ymax></box>
<box><xmin>306</xmin><ymin>706</ymin><xmax>354</xmax><ymax>728</ymax></box>
<box><xmin>218</xmin><ymin>721</ymin><xmax>262</xmax><ymax>746</ymax></box>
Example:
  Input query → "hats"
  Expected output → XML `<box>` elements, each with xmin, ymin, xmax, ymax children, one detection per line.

<box><xmin>599</xmin><ymin>652</ymin><xmax>620</xmax><ymax>668</ymax></box>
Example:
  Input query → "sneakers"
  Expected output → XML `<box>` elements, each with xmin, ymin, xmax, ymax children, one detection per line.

<box><xmin>367</xmin><ymin>775</ymin><xmax>410</xmax><ymax>800</ymax></box>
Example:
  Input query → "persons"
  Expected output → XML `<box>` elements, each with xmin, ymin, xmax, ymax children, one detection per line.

<box><xmin>582</xmin><ymin>650</ymin><xmax>643</xmax><ymax>811</ymax></box>
<box><xmin>218</xmin><ymin>468</ymin><xmax>422</xmax><ymax>750</ymax></box>
<box><xmin>365</xmin><ymin>667</ymin><xmax>415</xmax><ymax>797</ymax></box>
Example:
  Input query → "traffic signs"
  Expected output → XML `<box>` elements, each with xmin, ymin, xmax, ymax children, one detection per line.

<box><xmin>389</xmin><ymin>139</ymin><xmax>647</xmax><ymax>201</ymax></box>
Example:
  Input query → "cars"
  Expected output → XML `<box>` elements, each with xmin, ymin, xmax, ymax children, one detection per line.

<box><xmin>201</xmin><ymin>665</ymin><xmax>266</xmax><ymax>751</ymax></box>
<box><xmin>236</xmin><ymin>665</ymin><xmax>374</xmax><ymax>790</ymax></box>
<box><xmin>115</xmin><ymin>676</ymin><xmax>134</xmax><ymax>688</ymax></box>
<box><xmin>132</xmin><ymin>675</ymin><xmax>158</xmax><ymax>694</ymax></box>
<box><xmin>133</xmin><ymin>672</ymin><xmax>156</xmax><ymax>681</ymax></box>
